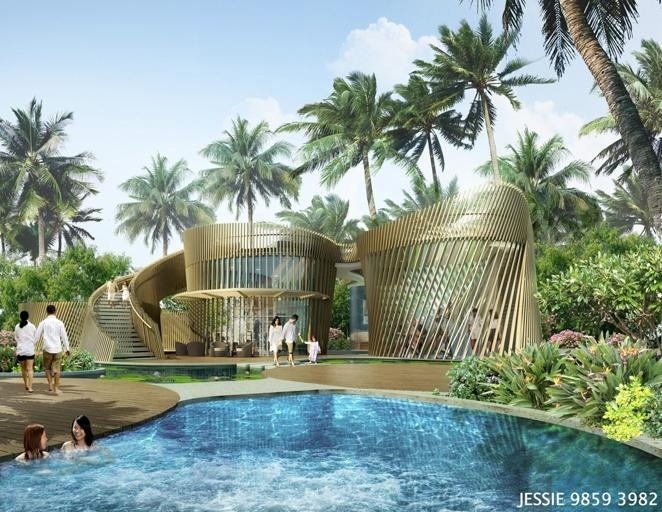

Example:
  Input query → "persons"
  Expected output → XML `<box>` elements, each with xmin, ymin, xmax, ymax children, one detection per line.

<box><xmin>396</xmin><ymin>298</ymin><xmax>504</xmax><ymax>359</ymax></box>
<box><xmin>119</xmin><ymin>281</ymin><xmax>131</xmax><ymax>309</ymax></box>
<box><xmin>63</xmin><ymin>414</ymin><xmax>99</xmax><ymax>454</ymax></box>
<box><xmin>14</xmin><ymin>424</ymin><xmax>51</xmax><ymax>460</ymax></box>
<box><xmin>34</xmin><ymin>305</ymin><xmax>70</xmax><ymax>394</ymax></box>
<box><xmin>282</xmin><ymin>314</ymin><xmax>303</xmax><ymax>367</ymax></box>
<box><xmin>14</xmin><ymin>309</ymin><xmax>38</xmax><ymax>392</ymax></box>
<box><xmin>269</xmin><ymin>316</ymin><xmax>284</xmax><ymax>365</ymax></box>
<box><xmin>107</xmin><ymin>277</ymin><xmax>120</xmax><ymax>308</ymax></box>
<box><xmin>304</xmin><ymin>336</ymin><xmax>321</xmax><ymax>364</ymax></box>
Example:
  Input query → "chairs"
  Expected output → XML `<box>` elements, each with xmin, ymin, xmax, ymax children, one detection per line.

<box><xmin>174</xmin><ymin>341</ymin><xmax>253</xmax><ymax>358</ymax></box>
